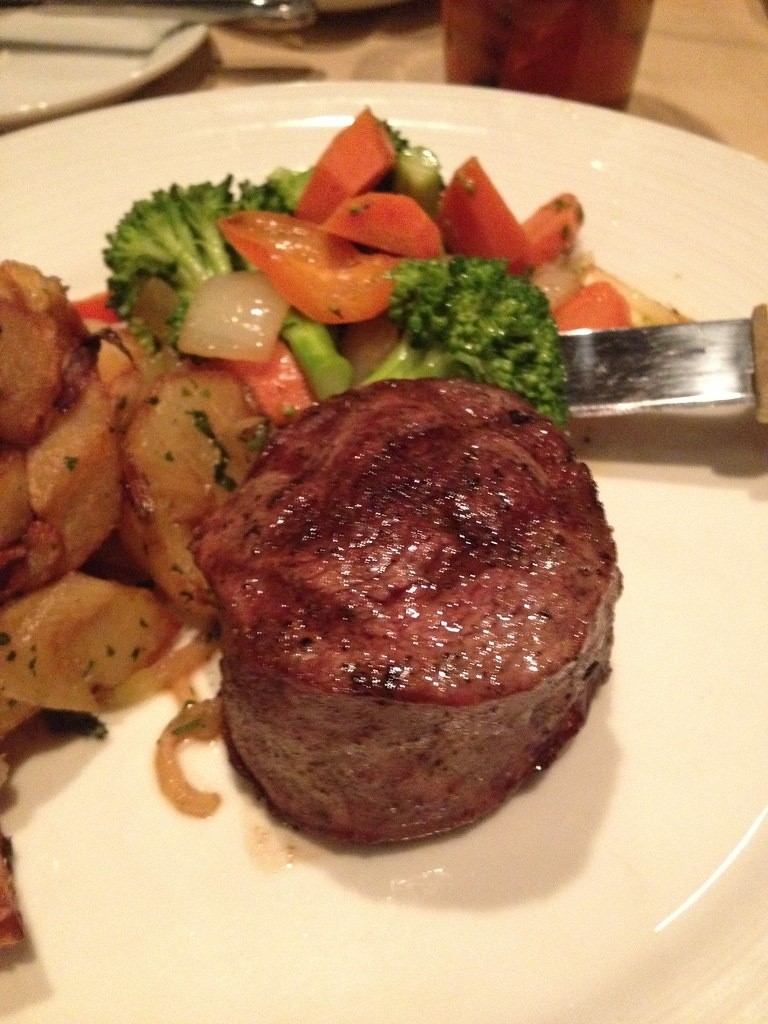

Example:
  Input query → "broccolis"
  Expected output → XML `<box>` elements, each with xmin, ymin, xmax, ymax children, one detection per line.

<box><xmin>105</xmin><ymin>121</ymin><xmax>569</xmax><ymax>430</ymax></box>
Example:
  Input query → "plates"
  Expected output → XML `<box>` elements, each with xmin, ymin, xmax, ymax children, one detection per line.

<box><xmin>0</xmin><ymin>80</ymin><xmax>768</xmax><ymax>1023</ymax></box>
<box><xmin>1</xmin><ymin>1</ymin><xmax>210</xmax><ymax>131</ymax></box>
